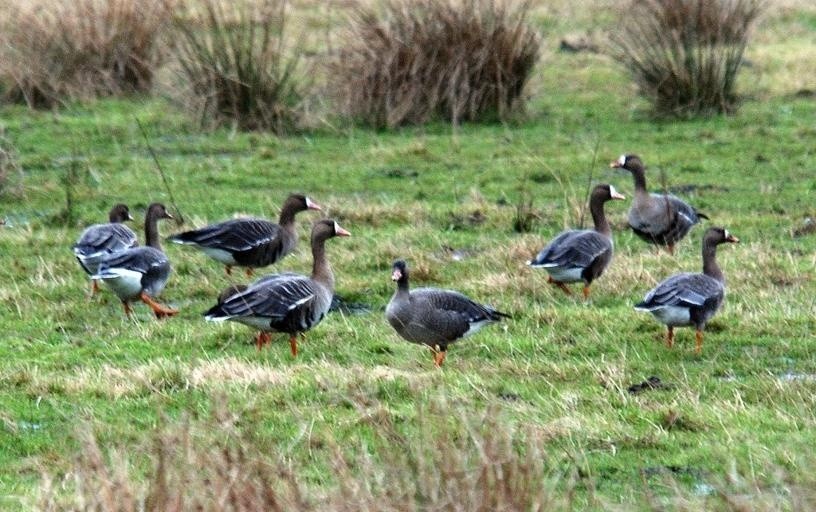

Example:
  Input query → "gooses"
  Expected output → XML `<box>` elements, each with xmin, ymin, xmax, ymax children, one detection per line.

<box><xmin>607</xmin><ymin>151</ymin><xmax>711</xmax><ymax>257</ymax></box>
<box><xmin>522</xmin><ymin>182</ymin><xmax>629</xmax><ymax>303</ymax></box>
<box><xmin>73</xmin><ymin>202</ymin><xmax>180</xmax><ymax>326</ymax></box>
<box><xmin>633</xmin><ymin>226</ymin><xmax>740</xmax><ymax>355</ymax></box>
<box><xmin>161</xmin><ymin>192</ymin><xmax>324</xmax><ymax>281</ymax></box>
<box><xmin>385</xmin><ymin>260</ymin><xmax>515</xmax><ymax>366</ymax></box>
<box><xmin>200</xmin><ymin>218</ymin><xmax>355</xmax><ymax>359</ymax></box>
